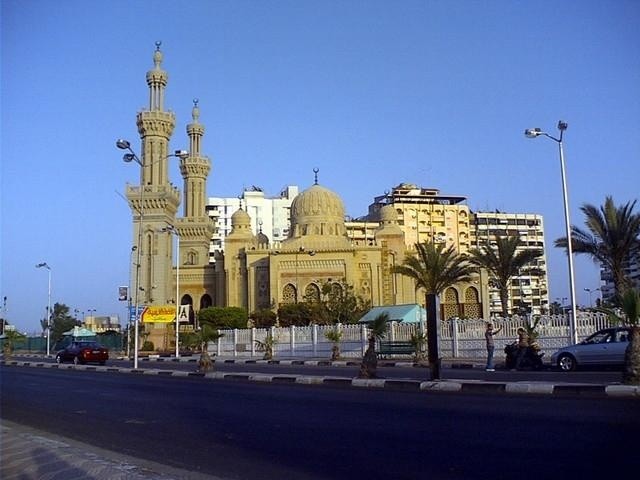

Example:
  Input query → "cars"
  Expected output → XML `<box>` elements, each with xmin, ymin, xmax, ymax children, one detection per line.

<box><xmin>551</xmin><ymin>326</ymin><xmax>633</xmax><ymax>372</ymax></box>
<box><xmin>56</xmin><ymin>341</ymin><xmax>109</xmax><ymax>365</ymax></box>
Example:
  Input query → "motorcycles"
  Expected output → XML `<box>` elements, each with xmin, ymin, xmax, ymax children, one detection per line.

<box><xmin>504</xmin><ymin>339</ymin><xmax>546</xmax><ymax>371</ymax></box>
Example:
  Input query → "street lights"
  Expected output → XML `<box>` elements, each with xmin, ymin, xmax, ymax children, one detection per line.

<box><xmin>524</xmin><ymin>120</ymin><xmax>578</xmax><ymax>345</ymax></box>
<box><xmin>73</xmin><ymin>306</ymin><xmax>96</xmax><ymax>325</ymax></box>
<box><xmin>35</xmin><ymin>262</ymin><xmax>52</xmax><ymax>358</ymax></box>
<box><xmin>583</xmin><ymin>287</ymin><xmax>601</xmax><ymax>307</ymax></box>
<box><xmin>295</xmin><ymin>246</ymin><xmax>315</xmax><ymax>304</ymax></box>
<box><xmin>116</xmin><ymin>138</ymin><xmax>189</xmax><ymax>370</ymax></box>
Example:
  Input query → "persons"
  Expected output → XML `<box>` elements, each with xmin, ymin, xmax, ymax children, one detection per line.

<box><xmin>509</xmin><ymin>328</ymin><xmax>531</xmax><ymax>373</ymax></box>
<box><xmin>485</xmin><ymin>322</ymin><xmax>503</xmax><ymax>373</ymax></box>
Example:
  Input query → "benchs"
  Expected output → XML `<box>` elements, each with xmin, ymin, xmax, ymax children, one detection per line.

<box><xmin>374</xmin><ymin>341</ymin><xmax>414</xmax><ymax>360</ymax></box>
